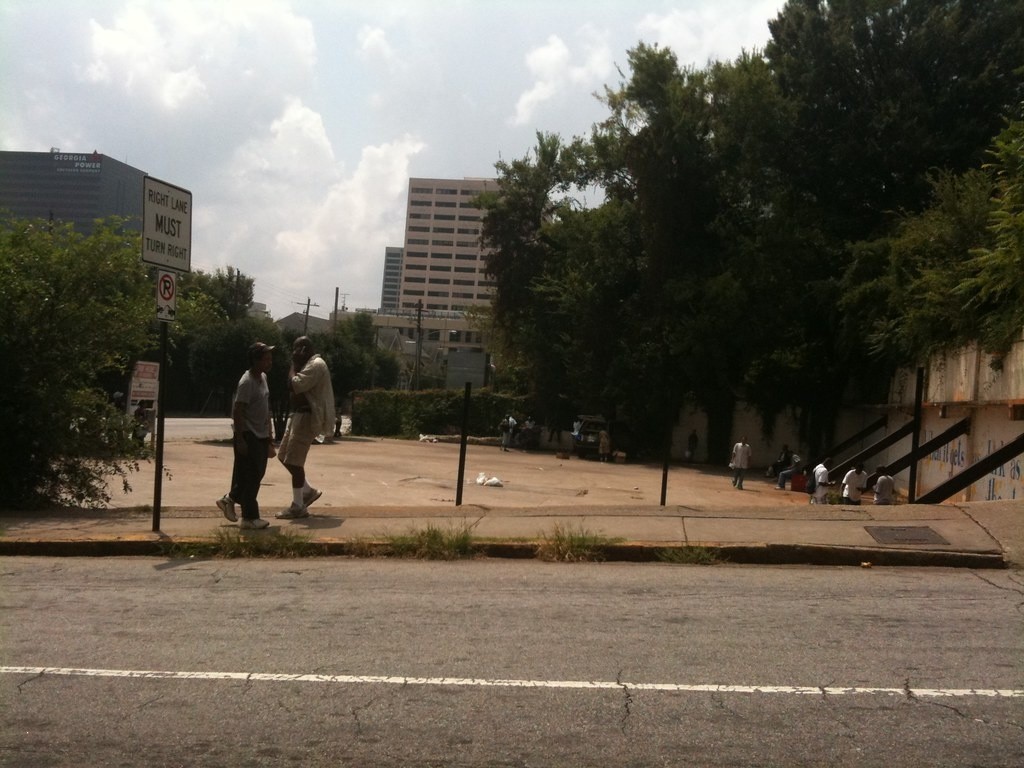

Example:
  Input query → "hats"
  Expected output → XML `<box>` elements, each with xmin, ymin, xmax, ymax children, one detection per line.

<box><xmin>249</xmin><ymin>346</ymin><xmax>275</xmax><ymax>357</ymax></box>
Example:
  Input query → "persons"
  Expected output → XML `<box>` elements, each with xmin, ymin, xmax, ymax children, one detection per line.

<box><xmin>687</xmin><ymin>429</ymin><xmax>699</xmax><ymax>463</ymax></box>
<box><xmin>599</xmin><ymin>430</ymin><xmax>610</xmax><ymax>462</ymax></box>
<box><xmin>839</xmin><ymin>464</ymin><xmax>871</xmax><ymax>504</ymax></box>
<box><xmin>730</xmin><ymin>435</ymin><xmax>752</xmax><ymax>490</ymax></box>
<box><xmin>132</xmin><ymin>400</ymin><xmax>146</xmax><ymax>447</ymax></box>
<box><xmin>772</xmin><ymin>445</ymin><xmax>801</xmax><ymax>490</ymax></box>
<box><xmin>498</xmin><ymin>413</ymin><xmax>514</xmax><ymax>452</ymax></box>
<box><xmin>217</xmin><ymin>341</ymin><xmax>276</xmax><ymax>529</ymax></box>
<box><xmin>275</xmin><ymin>335</ymin><xmax>337</xmax><ymax>518</ymax></box>
<box><xmin>812</xmin><ymin>457</ymin><xmax>836</xmax><ymax>500</ymax></box>
<box><xmin>872</xmin><ymin>466</ymin><xmax>895</xmax><ymax>505</ymax></box>
<box><xmin>525</xmin><ymin>416</ymin><xmax>535</xmax><ymax>429</ymax></box>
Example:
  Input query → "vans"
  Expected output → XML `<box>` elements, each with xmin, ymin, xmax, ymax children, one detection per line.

<box><xmin>571</xmin><ymin>415</ymin><xmax>610</xmax><ymax>459</ymax></box>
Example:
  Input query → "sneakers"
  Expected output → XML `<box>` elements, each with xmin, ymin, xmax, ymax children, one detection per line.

<box><xmin>216</xmin><ymin>493</ymin><xmax>238</xmax><ymax>522</ymax></box>
<box><xmin>240</xmin><ymin>517</ymin><xmax>270</xmax><ymax>530</ymax></box>
<box><xmin>274</xmin><ymin>501</ymin><xmax>309</xmax><ymax>519</ymax></box>
<box><xmin>303</xmin><ymin>488</ymin><xmax>322</xmax><ymax>507</ymax></box>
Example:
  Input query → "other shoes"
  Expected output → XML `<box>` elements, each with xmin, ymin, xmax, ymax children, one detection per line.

<box><xmin>775</xmin><ymin>486</ymin><xmax>785</xmax><ymax>491</ymax></box>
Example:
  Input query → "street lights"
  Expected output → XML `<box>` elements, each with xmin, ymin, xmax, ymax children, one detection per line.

<box><xmin>415</xmin><ymin>328</ymin><xmax>458</xmax><ymax>392</ymax></box>
